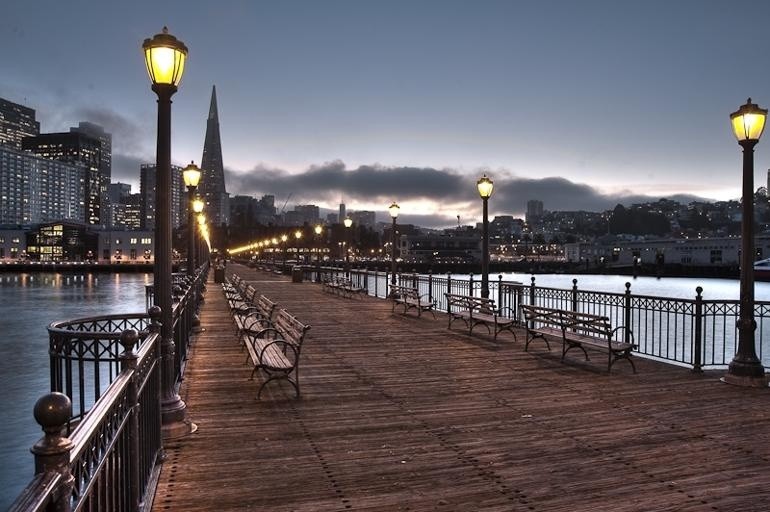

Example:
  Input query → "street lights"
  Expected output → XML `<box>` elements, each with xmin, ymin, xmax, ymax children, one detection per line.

<box><xmin>141</xmin><ymin>25</ymin><xmax>218</xmax><ymax>440</ymax></box>
<box><xmin>225</xmin><ymin>223</ymin><xmax>323</xmax><ymax>283</ymax></box>
<box><xmin>476</xmin><ymin>173</ymin><xmax>494</xmax><ymax>315</ymax></box>
<box><xmin>387</xmin><ymin>202</ymin><xmax>401</xmax><ymax>299</ymax></box>
<box><xmin>719</xmin><ymin>96</ymin><xmax>770</xmax><ymax>387</ymax></box>
<box><xmin>343</xmin><ymin>215</ymin><xmax>353</xmax><ymax>293</ymax></box>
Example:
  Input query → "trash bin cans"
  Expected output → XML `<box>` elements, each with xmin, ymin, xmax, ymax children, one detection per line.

<box><xmin>215</xmin><ymin>266</ymin><xmax>225</xmax><ymax>284</ymax></box>
<box><xmin>293</xmin><ymin>266</ymin><xmax>303</xmax><ymax>283</ymax></box>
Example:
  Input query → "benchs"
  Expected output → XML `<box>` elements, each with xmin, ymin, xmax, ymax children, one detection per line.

<box><xmin>321</xmin><ymin>274</ymin><xmax>363</xmax><ymax>299</ymax></box>
<box><xmin>221</xmin><ymin>273</ymin><xmax>312</xmax><ymax>400</ymax></box>
<box><xmin>389</xmin><ymin>284</ymin><xmax>437</xmax><ymax>320</ymax></box>
<box><xmin>519</xmin><ymin>304</ymin><xmax>639</xmax><ymax>374</ymax></box>
<box><xmin>444</xmin><ymin>293</ymin><xmax>518</xmax><ymax>342</ymax></box>
